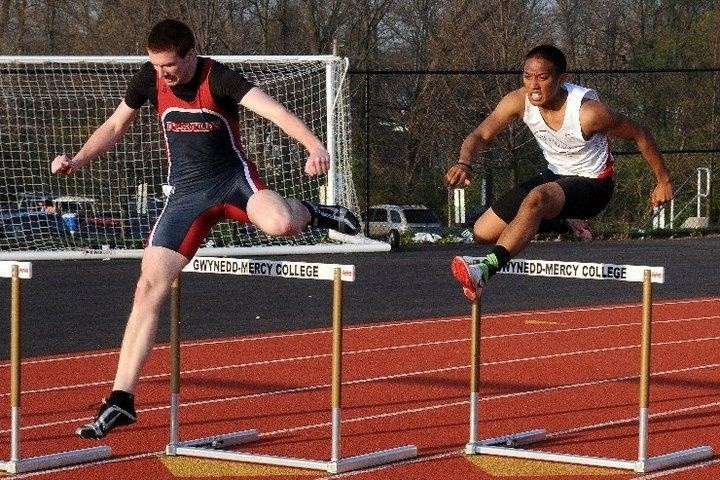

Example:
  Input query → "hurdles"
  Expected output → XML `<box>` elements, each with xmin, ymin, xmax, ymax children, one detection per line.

<box><xmin>165</xmin><ymin>256</ymin><xmax>417</xmax><ymax>475</ymax></box>
<box><xmin>0</xmin><ymin>259</ymin><xmax>110</xmax><ymax>475</ymax></box>
<box><xmin>462</xmin><ymin>256</ymin><xmax>712</xmax><ymax>473</ymax></box>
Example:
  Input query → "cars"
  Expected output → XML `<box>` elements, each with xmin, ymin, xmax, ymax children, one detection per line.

<box><xmin>361</xmin><ymin>202</ymin><xmax>442</xmax><ymax>248</ymax></box>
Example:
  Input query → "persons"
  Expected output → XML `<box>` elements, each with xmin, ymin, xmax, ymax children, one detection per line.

<box><xmin>51</xmin><ymin>19</ymin><xmax>363</xmax><ymax>439</ymax></box>
<box><xmin>439</xmin><ymin>46</ymin><xmax>675</xmax><ymax>302</ymax></box>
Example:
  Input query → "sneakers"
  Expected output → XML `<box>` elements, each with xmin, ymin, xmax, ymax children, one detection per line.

<box><xmin>567</xmin><ymin>218</ymin><xmax>596</xmax><ymax>244</ymax></box>
<box><xmin>73</xmin><ymin>389</ymin><xmax>138</xmax><ymax>439</ymax></box>
<box><xmin>301</xmin><ymin>200</ymin><xmax>361</xmax><ymax>237</ymax></box>
<box><xmin>451</xmin><ymin>252</ymin><xmax>499</xmax><ymax>303</ymax></box>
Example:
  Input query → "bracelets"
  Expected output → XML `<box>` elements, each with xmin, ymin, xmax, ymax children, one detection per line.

<box><xmin>456</xmin><ymin>157</ymin><xmax>474</xmax><ymax>173</ymax></box>
